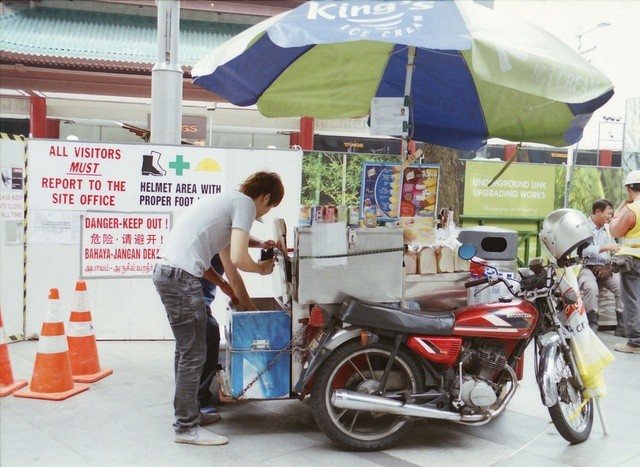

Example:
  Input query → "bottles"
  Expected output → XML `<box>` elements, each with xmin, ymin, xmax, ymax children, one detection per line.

<box><xmin>467</xmin><ymin>269</ymin><xmax>515</xmax><ymax>306</ymax></box>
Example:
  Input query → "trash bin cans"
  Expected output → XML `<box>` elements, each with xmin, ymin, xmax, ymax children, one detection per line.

<box><xmin>457</xmin><ymin>225</ymin><xmax>518</xmax><ymax>280</ymax></box>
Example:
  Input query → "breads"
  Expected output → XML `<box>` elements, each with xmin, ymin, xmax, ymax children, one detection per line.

<box><xmin>453</xmin><ymin>244</ymin><xmax>471</xmax><ymax>272</ymax></box>
<box><xmin>403</xmin><ymin>251</ymin><xmax>418</xmax><ymax>274</ymax></box>
<box><xmin>438</xmin><ymin>247</ymin><xmax>455</xmax><ymax>273</ymax></box>
<box><xmin>419</xmin><ymin>246</ymin><xmax>438</xmax><ymax>274</ymax></box>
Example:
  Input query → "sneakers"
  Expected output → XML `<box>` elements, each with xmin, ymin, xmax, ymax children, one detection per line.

<box><xmin>174</xmin><ymin>425</ymin><xmax>228</xmax><ymax>446</ymax></box>
<box><xmin>199</xmin><ymin>411</ymin><xmax>220</xmax><ymax>425</ymax></box>
<box><xmin>614</xmin><ymin>343</ymin><xmax>640</xmax><ymax>354</ymax></box>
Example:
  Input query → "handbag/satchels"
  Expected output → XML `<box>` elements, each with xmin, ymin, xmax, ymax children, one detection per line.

<box><xmin>586</xmin><ymin>265</ymin><xmax>612</xmax><ymax>280</ymax></box>
<box><xmin>610</xmin><ymin>256</ymin><xmax>633</xmax><ymax>273</ymax></box>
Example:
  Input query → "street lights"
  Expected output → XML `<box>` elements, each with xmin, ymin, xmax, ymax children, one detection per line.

<box><xmin>564</xmin><ymin>22</ymin><xmax>610</xmax><ymax>210</ymax></box>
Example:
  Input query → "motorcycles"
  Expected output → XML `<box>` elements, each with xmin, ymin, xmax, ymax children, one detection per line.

<box><xmin>293</xmin><ymin>236</ymin><xmax>594</xmax><ymax>452</ymax></box>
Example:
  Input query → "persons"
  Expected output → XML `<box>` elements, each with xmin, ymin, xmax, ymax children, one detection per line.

<box><xmin>198</xmin><ymin>233</ymin><xmax>278</xmax><ymax>413</ymax></box>
<box><xmin>608</xmin><ymin>168</ymin><xmax>640</xmax><ymax>354</ymax></box>
<box><xmin>151</xmin><ymin>170</ymin><xmax>284</xmax><ymax>446</ymax></box>
<box><xmin>577</xmin><ymin>199</ymin><xmax>625</xmax><ymax>337</ymax></box>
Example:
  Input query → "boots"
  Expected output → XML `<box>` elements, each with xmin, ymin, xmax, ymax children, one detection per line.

<box><xmin>587</xmin><ymin>309</ymin><xmax>599</xmax><ymax>335</ymax></box>
<box><xmin>615</xmin><ymin>311</ymin><xmax>625</xmax><ymax>337</ymax></box>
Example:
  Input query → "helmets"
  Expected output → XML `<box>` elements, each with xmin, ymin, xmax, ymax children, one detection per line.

<box><xmin>541</xmin><ymin>208</ymin><xmax>594</xmax><ymax>267</ymax></box>
<box><xmin>624</xmin><ymin>170</ymin><xmax>640</xmax><ymax>186</ymax></box>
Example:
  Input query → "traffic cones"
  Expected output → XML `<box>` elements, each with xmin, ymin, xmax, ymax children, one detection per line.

<box><xmin>66</xmin><ymin>280</ymin><xmax>113</xmax><ymax>382</ymax></box>
<box><xmin>0</xmin><ymin>308</ymin><xmax>28</xmax><ymax>397</ymax></box>
<box><xmin>13</xmin><ymin>288</ymin><xmax>89</xmax><ymax>400</ymax></box>
